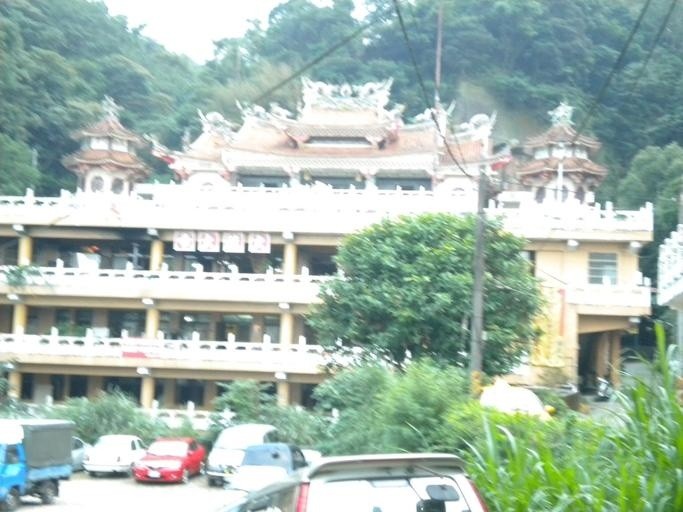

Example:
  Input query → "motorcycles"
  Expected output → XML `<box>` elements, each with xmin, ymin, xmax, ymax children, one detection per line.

<box><xmin>593</xmin><ymin>376</ymin><xmax>612</xmax><ymax>401</ymax></box>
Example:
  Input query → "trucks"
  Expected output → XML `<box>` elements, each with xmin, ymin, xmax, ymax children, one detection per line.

<box><xmin>0</xmin><ymin>418</ymin><xmax>78</xmax><ymax>511</ymax></box>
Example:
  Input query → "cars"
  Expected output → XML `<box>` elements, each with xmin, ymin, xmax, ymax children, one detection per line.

<box><xmin>70</xmin><ymin>436</ymin><xmax>87</xmax><ymax>472</ymax></box>
<box><xmin>131</xmin><ymin>434</ymin><xmax>207</xmax><ymax>483</ymax></box>
<box><xmin>83</xmin><ymin>435</ymin><xmax>148</xmax><ymax>477</ymax></box>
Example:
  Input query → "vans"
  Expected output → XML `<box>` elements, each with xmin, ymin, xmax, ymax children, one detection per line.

<box><xmin>199</xmin><ymin>423</ymin><xmax>279</xmax><ymax>487</ymax></box>
<box><xmin>222</xmin><ymin>443</ymin><xmax>306</xmax><ymax>501</ymax></box>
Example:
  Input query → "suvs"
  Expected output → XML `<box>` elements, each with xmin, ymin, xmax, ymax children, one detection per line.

<box><xmin>242</xmin><ymin>450</ymin><xmax>495</xmax><ymax>512</ymax></box>
